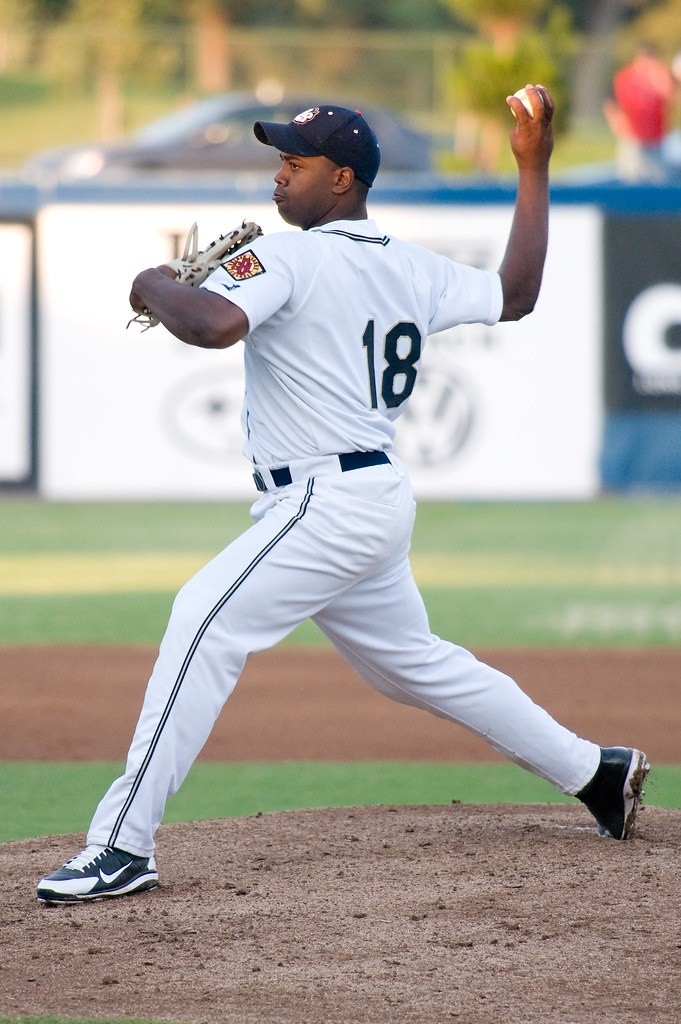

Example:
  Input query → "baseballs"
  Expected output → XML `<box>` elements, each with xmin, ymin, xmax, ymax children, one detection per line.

<box><xmin>509</xmin><ymin>87</ymin><xmax>546</xmax><ymax>119</ymax></box>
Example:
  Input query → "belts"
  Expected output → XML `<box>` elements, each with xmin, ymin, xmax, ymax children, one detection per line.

<box><xmin>252</xmin><ymin>451</ymin><xmax>392</xmax><ymax>492</ymax></box>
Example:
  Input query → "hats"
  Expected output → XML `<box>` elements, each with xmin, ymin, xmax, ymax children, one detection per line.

<box><xmin>253</xmin><ymin>106</ymin><xmax>381</xmax><ymax>187</ymax></box>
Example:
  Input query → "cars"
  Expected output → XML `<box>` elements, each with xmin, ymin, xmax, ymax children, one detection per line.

<box><xmin>21</xmin><ymin>89</ymin><xmax>452</xmax><ymax>183</ymax></box>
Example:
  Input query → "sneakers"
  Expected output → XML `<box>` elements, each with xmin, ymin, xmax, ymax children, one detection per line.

<box><xmin>574</xmin><ymin>746</ymin><xmax>649</xmax><ymax>840</ymax></box>
<box><xmin>36</xmin><ymin>844</ymin><xmax>159</xmax><ymax>904</ymax></box>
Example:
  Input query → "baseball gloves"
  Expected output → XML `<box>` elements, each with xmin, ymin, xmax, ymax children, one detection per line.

<box><xmin>131</xmin><ymin>221</ymin><xmax>264</xmax><ymax>328</ymax></box>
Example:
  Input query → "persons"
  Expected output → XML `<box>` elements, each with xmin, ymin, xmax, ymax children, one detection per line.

<box><xmin>34</xmin><ymin>81</ymin><xmax>654</xmax><ymax>905</ymax></box>
<box><xmin>613</xmin><ymin>41</ymin><xmax>680</xmax><ymax>168</ymax></box>
